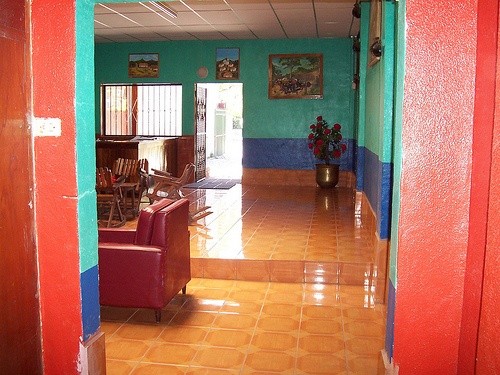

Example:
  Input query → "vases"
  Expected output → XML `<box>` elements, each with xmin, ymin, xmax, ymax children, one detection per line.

<box><xmin>315</xmin><ymin>162</ymin><xmax>340</xmax><ymax>188</ymax></box>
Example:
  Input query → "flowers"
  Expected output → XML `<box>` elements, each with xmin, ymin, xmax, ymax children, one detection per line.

<box><xmin>307</xmin><ymin>115</ymin><xmax>347</xmax><ymax>160</ymax></box>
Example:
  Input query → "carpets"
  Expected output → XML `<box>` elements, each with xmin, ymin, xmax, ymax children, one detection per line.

<box><xmin>183</xmin><ymin>177</ymin><xmax>240</xmax><ymax>189</ymax></box>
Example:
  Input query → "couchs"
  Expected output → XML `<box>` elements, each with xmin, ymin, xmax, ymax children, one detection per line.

<box><xmin>98</xmin><ymin>199</ymin><xmax>192</xmax><ymax>323</ymax></box>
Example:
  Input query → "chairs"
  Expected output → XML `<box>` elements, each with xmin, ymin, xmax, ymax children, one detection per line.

<box><xmin>95</xmin><ymin>158</ymin><xmax>214</xmax><ymax>229</ymax></box>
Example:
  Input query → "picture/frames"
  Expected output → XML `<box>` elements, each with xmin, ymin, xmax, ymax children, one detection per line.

<box><xmin>268</xmin><ymin>53</ymin><xmax>323</xmax><ymax>98</ymax></box>
<box><xmin>128</xmin><ymin>53</ymin><xmax>159</xmax><ymax>78</ymax></box>
<box><xmin>215</xmin><ymin>47</ymin><xmax>240</xmax><ymax>80</ymax></box>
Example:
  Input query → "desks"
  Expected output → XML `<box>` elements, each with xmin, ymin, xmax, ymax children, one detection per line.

<box><xmin>102</xmin><ymin>181</ymin><xmax>137</xmax><ymax>218</ymax></box>
